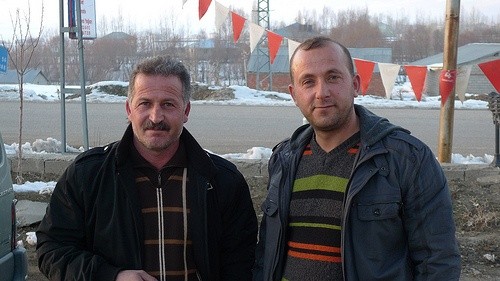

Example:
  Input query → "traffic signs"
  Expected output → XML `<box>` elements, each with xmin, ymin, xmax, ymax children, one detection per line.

<box><xmin>68</xmin><ymin>0</ymin><xmax>97</xmax><ymax>39</ymax></box>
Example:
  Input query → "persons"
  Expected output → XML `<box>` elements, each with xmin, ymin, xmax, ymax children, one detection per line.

<box><xmin>253</xmin><ymin>35</ymin><xmax>462</xmax><ymax>281</ymax></box>
<box><xmin>34</xmin><ymin>56</ymin><xmax>258</xmax><ymax>281</ymax></box>
